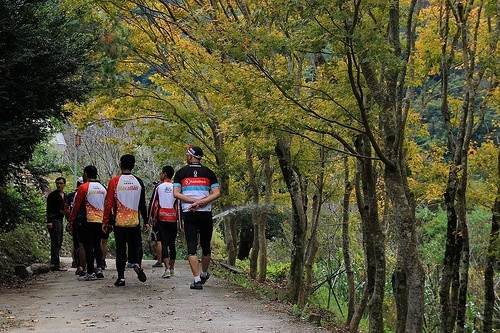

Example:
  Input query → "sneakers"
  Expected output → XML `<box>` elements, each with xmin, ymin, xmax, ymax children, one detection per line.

<box><xmin>170</xmin><ymin>268</ymin><xmax>174</xmax><ymax>276</ymax></box>
<box><xmin>78</xmin><ymin>271</ymin><xmax>97</xmax><ymax>280</ymax></box>
<box><xmin>162</xmin><ymin>269</ymin><xmax>170</xmax><ymax>278</ymax></box>
<box><xmin>97</xmin><ymin>268</ymin><xmax>104</xmax><ymax>278</ymax></box>
<box><xmin>200</xmin><ymin>272</ymin><xmax>210</xmax><ymax>284</ymax></box>
<box><xmin>190</xmin><ymin>279</ymin><xmax>202</xmax><ymax>289</ymax></box>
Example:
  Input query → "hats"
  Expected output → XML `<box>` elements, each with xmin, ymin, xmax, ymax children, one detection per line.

<box><xmin>78</xmin><ymin>176</ymin><xmax>84</xmax><ymax>183</ymax></box>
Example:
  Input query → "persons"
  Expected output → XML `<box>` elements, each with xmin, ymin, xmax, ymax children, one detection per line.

<box><xmin>66</xmin><ymin>164</ymin><xmax>112</xmax><ymax>280</ymax></box>
<box><xmin>173</xmin><ymin>145</ymin><xmax>221</xmax><ymax>290</ymax></box>
<box><xmin>102</xmin><ymin>154</ymin><xmax>149</xmax><ymax>286</ymax></box>
<box><xmin>150</xmin><ymin>209</ymin><xmax>171</xmax><ymax>267</ymax></box>
<box><xmin>127</xmin><ymin>245</ymin><xmax>134</xmax><ymax>268</ymax></box>
<box><xmin>148</xmin><ymin>166</ymin><xmax>183</xmax><ymax>277</ymax></box>
<box><xmin>47</xmin><ymin>177</ymin><xmax>67</xmax><ymax>271</ymax></box>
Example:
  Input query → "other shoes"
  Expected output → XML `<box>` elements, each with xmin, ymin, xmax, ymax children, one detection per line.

<box><xmin>80</xmin><ymin>271</ymin><xmax>87</xmax><ymax>277</ymax></box>
<box><xmin>76</xmin><ymin>267</ymin><xmax>83</xmax><ymax>275</ymax></box>
<box><xmin>135</xmin><ymin>263</ymin><xmax>146</xmax><ymax>282</ymax></box>
<box><xmin>114</xmin><ymin>278</ymin><xmax>125</xmax><ymax>286</ymax></box>
<box><xmin>152</xmin><ymin>261</ymin><xmax>163</xmax><ymax>267</ymax></box>
<box><xmin>103</xmin><ymin>260</ymin><xmax>107</xmax><ymax>270</ymax></box>
<box><xmin>127</xmin><ymin>263</ymin><xmax>134</xmax><ymax>268</ymax></box>
